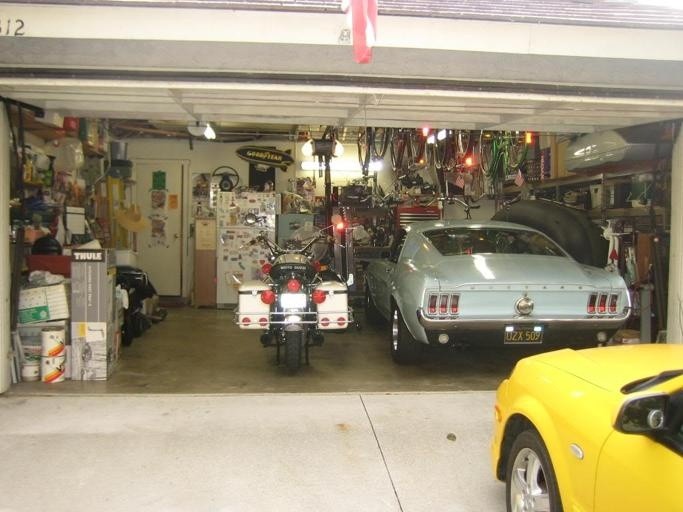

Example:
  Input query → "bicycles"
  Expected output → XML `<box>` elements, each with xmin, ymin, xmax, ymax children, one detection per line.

<box><xmin>354</xmin><ymin>125</ymin><xmax>527</xmax><ymax>217</ymax></box>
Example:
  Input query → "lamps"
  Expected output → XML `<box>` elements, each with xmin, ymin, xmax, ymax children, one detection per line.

<box><xmin>187</xmin><ymin>120</ymin><xmax>216</xmax><ymax>140</ymax></box>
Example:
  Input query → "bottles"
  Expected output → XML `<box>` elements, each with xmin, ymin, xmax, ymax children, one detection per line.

<box><xmin>197</xmin><ymin>201</ymin><xmax>202</xmax><ymax>217</ymax></box>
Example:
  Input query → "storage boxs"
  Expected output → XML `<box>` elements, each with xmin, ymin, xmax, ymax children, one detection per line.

<box><xmin>71</xmin><ymin>248</ymin><xmax>117</xmax><ymax>382</ymax></box>
<box><xmin>18</xmin><ymin>280</ymin><xmax>71</xmax><ymax>325</ymax></box>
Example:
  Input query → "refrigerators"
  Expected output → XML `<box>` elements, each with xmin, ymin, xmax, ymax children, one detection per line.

<box><xmin>215</xmin><ymin>191</ymin><xmax>277</xmax><ymax>310</ymax></box>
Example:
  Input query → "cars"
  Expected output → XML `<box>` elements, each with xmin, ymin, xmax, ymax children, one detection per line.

<box><xmin>487</xmin><ymin>343</ymin><xmax>682</xmax><ymax>512</ymax></box>
<box><xmin>363</xmin><ymin>217</ymin><xmax>631</xmax><ymax>365</ymax></box>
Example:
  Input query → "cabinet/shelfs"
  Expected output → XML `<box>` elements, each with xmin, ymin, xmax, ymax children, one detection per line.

<box><xmin>490</xmin><ymin>159</ymin><xmax>670</xmax><ymax>232</ymax></box>
<box><xmin>194</xmin><ymin>218</ymin><xmax>217</xmax><ymax>309</ymax></box>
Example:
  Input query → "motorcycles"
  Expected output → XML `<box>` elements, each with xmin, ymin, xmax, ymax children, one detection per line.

<box><xmin>234</xmin><ymin>212</ymin><xmax>356</xmax><ymax>368</ymax></box>
